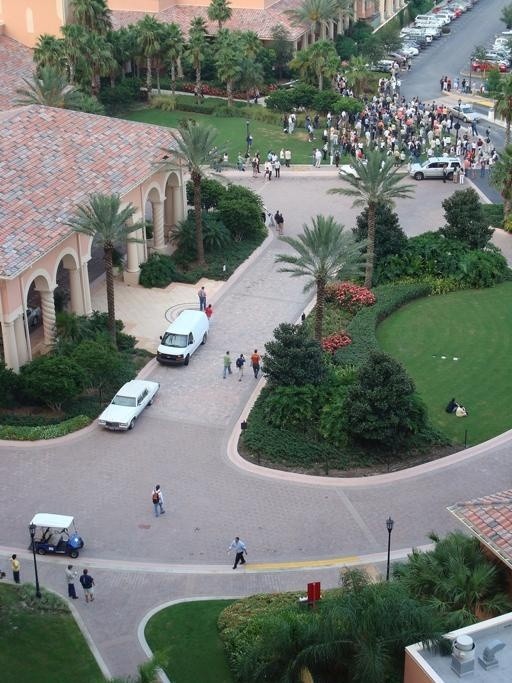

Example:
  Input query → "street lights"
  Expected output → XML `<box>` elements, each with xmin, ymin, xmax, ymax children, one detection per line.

<box><xmin>246</xmin><ymin>118</ymin><xmax>249</xmax><ymax>154</ymax></box>
<box><xmin>29</xmin><ymin>521</ymin><xmax>41</xmax><ymax>597</ymax></box>
<box><xmin>456</xmin><ymin>98</ymin><xmax>462</xmax><ymax>138</ymax></box>
<box><xmin>386</xmin><ymin>516</ymin><xmax>393</xmax><ymax>580</ymax></box>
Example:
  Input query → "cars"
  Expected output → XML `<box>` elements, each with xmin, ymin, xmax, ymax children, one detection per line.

<box><xmin>364</xmin><ymin>0</ymin><xmax>511</xmax><ymax>72</ymax></box>
<box><xmin>98</xmin><ymin>379</ymin><xmax>160</xmax><ymax>430</ymax></box>
<box><xmin>447</xmin><ymin>103</ymin><xmax>481</xmax><ymax>122</ymax></box>
<box><xmin>24</xmin><ymin>301</ymin><xmax>41</xmax><ymax>328</ymax></box>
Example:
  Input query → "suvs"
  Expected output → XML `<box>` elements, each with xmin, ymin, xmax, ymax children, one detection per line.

<box><xmin>337</xmin><ymin>158</ymin><xmax>389</xmax><ymax>180</ymax></box>
<box><xmin>408</xmin><ymin>156</ymin><xmax>465</xmax><ymax>180</ymax></box>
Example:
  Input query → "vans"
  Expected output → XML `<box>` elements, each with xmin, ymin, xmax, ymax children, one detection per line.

<box><xmin>157</xmin><ymin>309</ymin><xmax>210</xmax><ymax>366</ymax></box>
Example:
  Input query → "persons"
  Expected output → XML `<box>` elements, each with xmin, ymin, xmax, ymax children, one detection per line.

<box><xmin>455</xmin><ymin>405</ymin><xmax>468</xmax><ymax>417</ymax></box>
<box><xmin>250</xmin><ymin>349</ymin><xmax>262</xmax><ymax>379</ymax></box>
<box><xmin>236</xmin><ymin>354</ymin><xmax>246</xmax><ymax>381</ymax></box>
<box><xmin>274</xmin><ymin>210</ymin><xmax>284</xmax><ymax>235</ymax></box>
<box><xmin>445</xmin><ymin>397</ymin><xmax>458</xmax><ymax>414</ymax></box>
<box><xmin>209</xmin><ymin>55</ymin><xmax>500</xmax><ymax>185</ymax></box>
<box><xmin>198</xmin><ymin>287</ymin><xmax>207</xmax><ymax>311</ymax></box>
<box><xmin>203</xmin><ymin>304</ymin><xmax>213</xmax><ymax>321</ymax></box>
<box><xmin>226</xmin><ymin>536</ymin><xmax>247</xmax><ymax>569</ymax></box>
<box><xmin>79</xmin><ymin>568</ymin><xmax>96</xmax><ymax>603</ymax></box>
<box><xmin>222</xmin><ymin>351</ymin><xmax>233</xmax><ymax>379</ymax></box>
<box><xmin>11</xmin><ymin>554</ymin><xmax>21</xmax><ymax>584</ymax></box>
<box><xmin>65</xmin><ymin>565</ymin><xmax>79</xmax><ymax>599</ymax></box>
<box><xmin>152</xmin><ymin>484</ymin><xmax>165</xmax><ymax>517</ymax></box>
